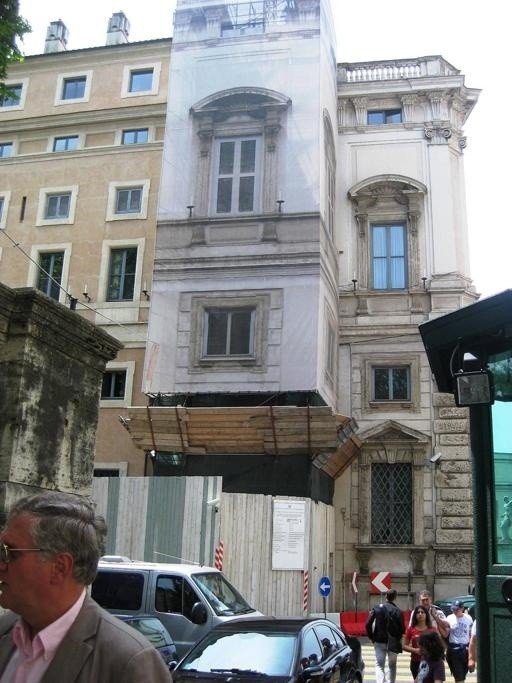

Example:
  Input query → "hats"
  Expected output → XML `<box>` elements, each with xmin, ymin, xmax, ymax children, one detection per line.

<box><xmin>451</xmin><ymin>600</ymin><xmax>464</xmax><ymax>610</ymax></box>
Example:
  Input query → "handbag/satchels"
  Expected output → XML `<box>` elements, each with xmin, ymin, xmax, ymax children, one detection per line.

<box><xmin>387</xmin><ymin>614</ymin><xmax>403</xmax><ymax>653</ymax></box>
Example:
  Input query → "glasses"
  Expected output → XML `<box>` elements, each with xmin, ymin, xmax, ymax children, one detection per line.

<box><xmin>1</xmin><ymin>542</ymin><xmax>49</xmax><ymax>563</ymax></box>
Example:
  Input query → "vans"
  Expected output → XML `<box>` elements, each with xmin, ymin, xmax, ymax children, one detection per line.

<box><xmin>92</xmin><ymin>561</ymin><xmax>264</xmax><ymax>673</ymax></box>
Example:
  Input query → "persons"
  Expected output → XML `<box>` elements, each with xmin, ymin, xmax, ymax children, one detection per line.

<box><xmin>499</xmin><ymin>496</ymin><xmax>512</xmax><ymax>540</ymax></box>
<box><xmin>309</xmin><ymin>653</ymin><xmax>319</xmax><ymax>664</ymax></box>
<box><xmin>366</xmin><ymin>587</ymin><xmax>477</xmax><ymax>682</ymax></box>
<box><xmin>301</xmin><ymin>656</ymin><xmax>310</xmax><ymax>669</ymax></box>
<box><xmin>320</xmin><ymin>637</ymin><xmax>339</xmax><ymax>659</ymax></box>
<box><xmin>0</xmin><ymin>489</ymin><xmax>176</xmax><ymax>682</ymax></box>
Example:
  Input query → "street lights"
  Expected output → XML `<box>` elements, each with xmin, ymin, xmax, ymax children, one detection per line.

<box><xmin>419</xmin><ymin>289</ymin><xmax>512</xmax><ymax>682</ymax></box>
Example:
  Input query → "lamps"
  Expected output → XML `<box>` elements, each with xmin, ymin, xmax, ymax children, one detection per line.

<box><xmin>352</xmin><ymin>272</ymin><xmax>357</xmax><ymax>291</ymax></box>
<box><xmin>142</xmin><ymin>281</ymin><xmax>149</xmax><ymax>300</ymax></box>
<box><xmin>66</xmin><ymin>286</ymin><xmax>72</xmax><ymax>300</ymax></box>
<box><xmin>84</xmin><ymin>284</ymin><xmax>91</xmax><ymax>303</ymax></box>
<box><xmin>421</xmin><ymin>270</ymin><xmax>427</xmax><ymax>289</ymax></box>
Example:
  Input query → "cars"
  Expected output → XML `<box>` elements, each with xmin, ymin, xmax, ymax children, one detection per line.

<box><xmin>432</xmin><ymin>585</ymin><xmax>476</xmax><ymax>617</ymax></box>
<box><xmin>168</xmin><ymin>618</ymin><xmax>364</xmax><ymax>683</ymax></box>
